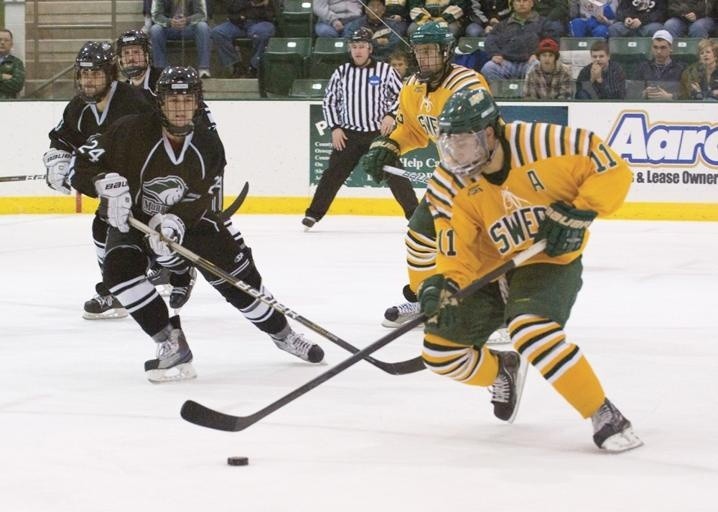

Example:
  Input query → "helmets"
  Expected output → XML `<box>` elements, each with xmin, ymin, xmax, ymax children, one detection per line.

<box><xmin>406</xmin><ymin>22</ymin><xmax>456</xmax><ymax>83</ymax></box>
<box><xmin>435</xmin><ymin>88</ymin><xmax>501</xmax><ymax>177</ymax></box>
<box><xmin>116</xmin><ymin>30</ymin><xmax>151</xmax><ymax>79</ymax></box>
<box><xmin>154</xmin><ymin>65</ymin><xmax>203</xmax><ymax>136</ymax></box>
<box><xmin>73</xmin><ymin>41</ymin><xmax>116</xmax><ymax>104</ymax></box>
<box><xmin>349</xmin><ymin>28</ymin><xmax>374</xmax><ymax>43</ymax></box>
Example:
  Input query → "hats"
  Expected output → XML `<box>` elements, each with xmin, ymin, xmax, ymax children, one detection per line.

<box><xmin>536</xmin><ymin>38</ymin><xmax>560</xmax><ymax>54</ymax></box>
<box><xmin>651</xmin><ymin>30</ymin><xmax>672</xmax><ymax>45</ymax></box>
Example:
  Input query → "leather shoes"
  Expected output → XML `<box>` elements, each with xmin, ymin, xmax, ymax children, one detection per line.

<box><xmin>232</xmin><ymin>62</ymin><xmax>246</xmax><ymax>77</ymax></box>
<box><xmin>246</xmin><ymin>65</ymin><xmax>257</xmax><ymax>78</ymax></box>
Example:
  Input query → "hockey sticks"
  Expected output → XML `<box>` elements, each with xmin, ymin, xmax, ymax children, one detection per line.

<box><xmin>50</xmin><ymin>130</ymin><xmax>248</xmax><ymax>219</ymax></box>
<box><xmin>180</xmin><ymin>239</ymin><xmax>548</xmax><ymax>431</ymax></box>
<box><xmin>125</xmin><ymin>212</ymin><xmax>426</xmax><ymax>375</ymax></box>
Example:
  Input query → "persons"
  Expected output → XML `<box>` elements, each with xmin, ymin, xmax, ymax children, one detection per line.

<box><xmin>311</xmin><ymin>1</ymin><xmax>718</xmax><ymax>103</ymax></box>
<box><xmin>407</xmin><ymin>90</ymin><xmax>644</xmax><ymax>453</ymax></box>
<box><xmin>303</xmin><ymin>31</ymin><xmax>419</xmax><ymax>227</ymax></box>
<box><xmin>116</xmin><ymin>30</ymin><xmax>178</xmax><ymax>286</ymax></box>
<box><xmin>141</xmin><ymin>1</ymin><xmax>286</xmax><ymax>77</ymax></box>
<box><xmin>1</xmin><ymin>29</ymin><xmax>25</xmax><ymax>100</ymax></box>
<box><xmin>43</xmin><ymin>41</ymin><xmax>196</xmax><ymax>320</ymax></box>
<box><xmin>364</xmin><ymin>23</ymin><xmax>498</xmax><ymax>327</ymax></box>
<box><xmin>69</xmin><ymin>67</ymin><xmax>328</xmax><ymax>381</ymax></box>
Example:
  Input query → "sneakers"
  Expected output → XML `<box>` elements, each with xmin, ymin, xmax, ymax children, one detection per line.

<box><xmin>84</xmin><ymin>282</ymin><xmax>123</xmax><ymax>313</ymax></box>
<box><xmin>591</xmin><ymin>397</ymin><xmax>631</xmax><ymax>448</ymax></box>
<box><xmin>145</xmin><ymin>315</ymin><xmax>193</xmax><ymax>370</ymax></box>
<box><xmin>302</xmin><ymin>215</ymin><xmax>316</xmax><ymax>227</ymax></box>
<box><xmin>198</xmin><ymin>69</ymin><xmax>210</xmax><ymax>78</ymax></box>
<box><xmin>489</xmin><ymin>349</ymin><xmax>520</xmax><ymax>420</ymax></box>
<box><xmin>270</xmin><ymin>327</ymin><xmax>324</xmax><ymax>362</ymax></box>
<box><xmin>148</xmin><ymin>264</ymin><xmax>196</xmax><ymax>309</ymax></box>
<box><xmin>384</xmin><ymin>301</ymin><xmax>421</xmax><ymax>324</ymax></box>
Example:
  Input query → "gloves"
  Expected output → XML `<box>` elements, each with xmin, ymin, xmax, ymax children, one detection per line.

<box><xmin>363</xmin><ymin>136</ymin><xmax>400</xmax><ymax>186</ymax></box>
<box><xmin>43</xmin><ymin>147</ymin><xmax>73</xmax><ymax>195</ymax></box>
<box><xmin>92</xmin><ymin>172</ymin><xmax>133</xmax><ymax>234</ymax></box>
<box><xmin>145</xmin><ymin>212</ymin><xmax>186</xmax><ymax>257</ymax></box>
<box><xmin>416</xmin><ymin>274</ymin><xmax>460</xmax><ymax>334</ymax></box>
<box><xmin>534</xmin><ymin>201</ymin><xmax>598</xmax><ymax>258</ymax></box>
